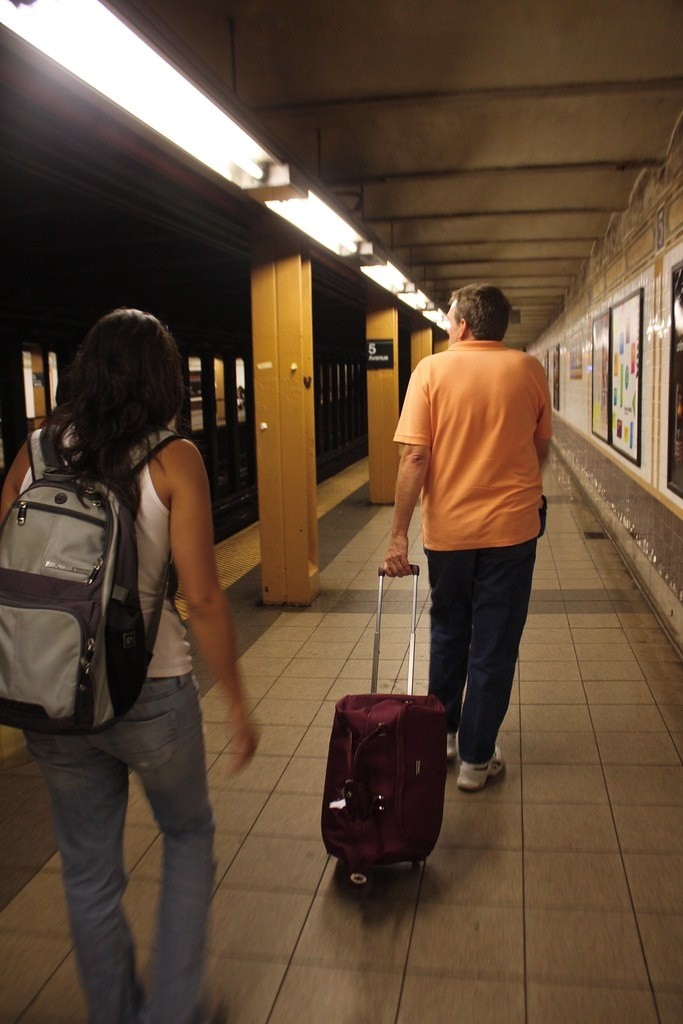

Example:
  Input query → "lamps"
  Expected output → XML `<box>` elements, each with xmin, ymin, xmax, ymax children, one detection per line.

<box><xmin>0</xmin><ymin>0</ymin><xmax>451</xmax><ymax>333</ymax></box>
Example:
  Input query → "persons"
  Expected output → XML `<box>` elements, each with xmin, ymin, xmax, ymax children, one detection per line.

<box><xmin>0</xmin><ymin>307</ymin><xmax>260</xmax><ymax>1024</ymax></box>
<box><xmin>382</xmin><ymin>283</ymin><xmax>554</xmax><ymax>793</ymax></box>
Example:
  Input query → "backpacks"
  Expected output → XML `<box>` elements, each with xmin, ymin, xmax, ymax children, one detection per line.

<box><xmin>0</xmin><ymin>426</ymin><xmax>190</xmax><ymax>732</ymax></box>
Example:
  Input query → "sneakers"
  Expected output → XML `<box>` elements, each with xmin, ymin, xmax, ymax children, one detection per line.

<box><xmin>446</xmin><ymin>733</ymin><xmax>459</xmax><ymax>758</ymax></box>
<box><xmin>457</xmin><ymin>746</ymin><xmax>504</xmax><ymax>790</ymax></box>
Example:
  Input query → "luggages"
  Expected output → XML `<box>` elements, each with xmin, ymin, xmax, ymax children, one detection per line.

<box><xmin>320</xmin><ymin>565</ymin><xmax>447</xmax><ymax>884</ymax></box>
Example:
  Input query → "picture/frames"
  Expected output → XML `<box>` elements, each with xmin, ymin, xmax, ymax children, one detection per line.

<box><xmin>553</xmin><ymin>342</ymin><xmax>560</xmax><ymax>412</ymax></box>
<box><xmin>544</xmin><ymin>349</ymin><xmax>549</xmax><ymax>380</ymax></box>
<box><xmin>587</xmin><ymin>287</ymin><xmax>644</xmax><ymax>468</ymax></box>
<box><xmin>667</xmin><ymin>259</ymin><xmax>683</xmax><ymax>499</ymax></box>
